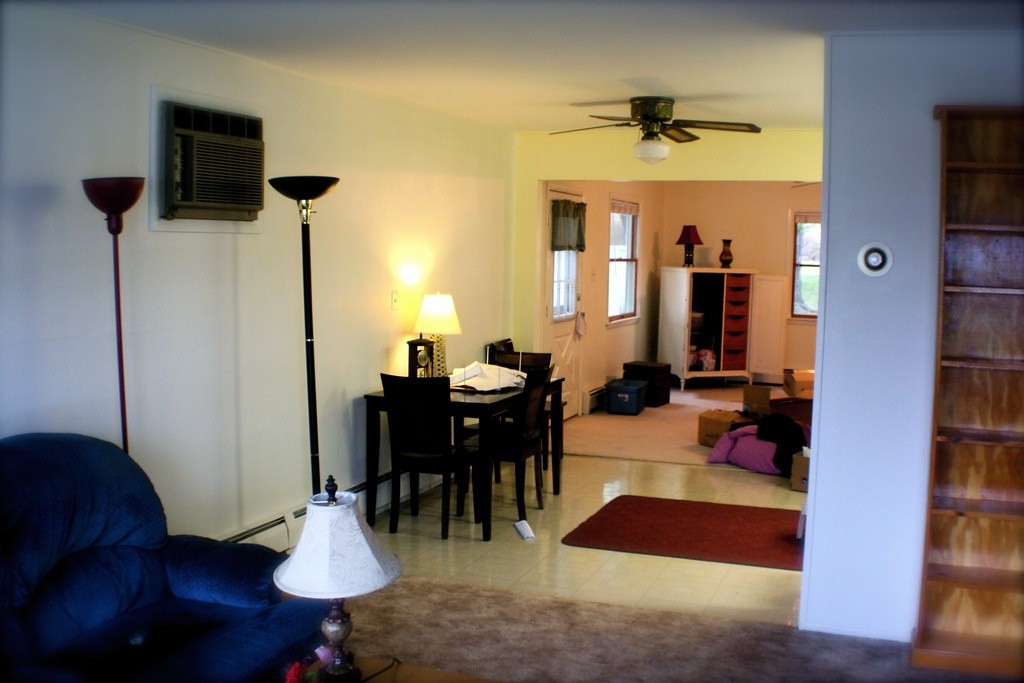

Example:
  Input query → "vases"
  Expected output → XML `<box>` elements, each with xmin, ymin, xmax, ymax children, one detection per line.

<box><xmin>719</xmin><ymin>239</ymin><xmax>733</xmax><ymax>268</ymax></box>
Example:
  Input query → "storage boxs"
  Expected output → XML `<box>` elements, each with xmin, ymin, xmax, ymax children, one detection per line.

<box><xmin>698</xmin><ymin>407</ymin><xmax>739</xmax><ymax>448</ymax></box>
<box><xmin>604</xmin><ymin>379</ymin><xmax>648</xmax><ymax>416</ymax></box>
<box><xmin>623</xmin><ymin>360</ymin><xmax>671</xmax><ymax>408</ymax></box>
<box><xmin>791</xmin><ymin>451</ymin><xmax>810</xmax><ymax>493</ymax></box>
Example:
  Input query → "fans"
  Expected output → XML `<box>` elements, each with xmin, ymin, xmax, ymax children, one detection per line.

<box><xmin>548</xmin><ymin>96</ymin><xmax>762</xmax><ymax>144</ymax></box>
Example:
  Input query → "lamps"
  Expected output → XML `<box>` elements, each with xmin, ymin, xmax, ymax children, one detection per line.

<box><xmin>631</xmin><ymin>141</ymin><xmax>670</xmax><ymax>164</ymax></box>
<box><xmin>82</xmin><ymin>175</ymin><xmax>148</xmax><ymax>459</ymax></box>
<box><xmin>675</xmin><ymin>224</ymin><xmax>704</xmax><ymax>267</ymax></box>
<box><xmin>266</xmin><ymin>175</ymin><xmax>343</xmax><ymax>496</ymax></box>
<box><xmin>273</xmin><ymin>474</ymin><xmax>406</xmax><ymax>683</ymax></box>
<box><xmin>413</xmin><ymin>291</ymin><xmax>462</xmax><ymax>377</ymax></box>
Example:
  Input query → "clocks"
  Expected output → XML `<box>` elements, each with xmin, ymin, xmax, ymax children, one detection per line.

<box><xmin>408</xmin><ymin>333</ymin><xmax>436</xmax><ymax>377</ymax></box>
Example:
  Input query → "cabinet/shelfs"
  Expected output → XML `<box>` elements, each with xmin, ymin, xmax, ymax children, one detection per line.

<box><xmin>657</xmin><ymin>265</ymin><xmax>755</xmax><ymax>392</ymax></box>
<box><xmin>905</xmin><ymin>105</ymin><xmax>1024</xmax><ymax>681</ymax></box>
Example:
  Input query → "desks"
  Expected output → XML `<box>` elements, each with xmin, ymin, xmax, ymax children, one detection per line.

<box><xmin>364</xmin><ymin>371</ymin><xmax>567</xmax><ymax>524</ymax></box>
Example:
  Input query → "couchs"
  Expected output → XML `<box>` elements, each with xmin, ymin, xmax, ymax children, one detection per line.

<box><xmin>0</xmin><ymin>432</ymin><xmax>334</xmax><ymax>683</ymax></box>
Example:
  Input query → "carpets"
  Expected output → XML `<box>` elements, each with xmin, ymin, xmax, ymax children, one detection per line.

<box><xmin>561</xmin><ymin>495</ymin><xmax>805</xmax><ymax>574</ymax></box>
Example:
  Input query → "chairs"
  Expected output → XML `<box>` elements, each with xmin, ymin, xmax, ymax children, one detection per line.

<box><xmin>380</xmin><ymin>338</ymin><xmax>570</xmax><ymax>539</ymax></box>
